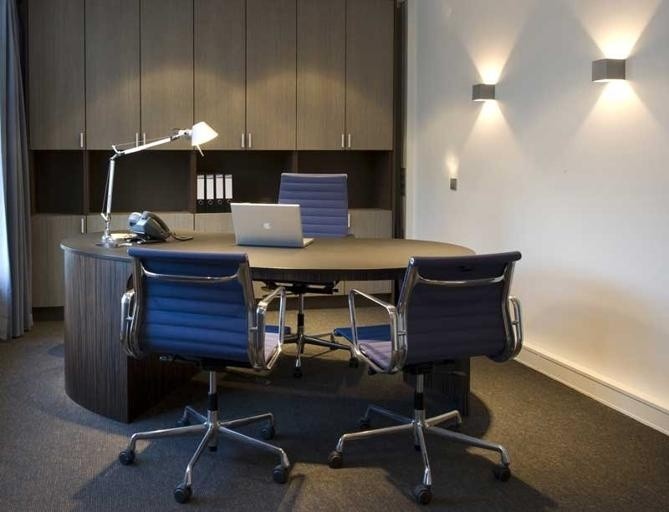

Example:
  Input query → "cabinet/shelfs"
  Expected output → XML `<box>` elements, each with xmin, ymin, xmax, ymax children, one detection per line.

<box><xmin>28</xmin><ymin>213</ymin><xmax>87</xmax><ymax>324</ymax></box>
<box><xmin>81</xmin><ymin>1</ymin><xmax>196</xmax><ymax>155</ymax></box>
<box><xmin>190</xmin><ymin>0</ymin><xmax>298</xmax><ymax>160</ymax></box>
<box><xmin>193</xmin><ymin>211</ymin><xmax>302</xmax><ymax>301</ymax></box>
<box><xmin>13</xmin><ymin>1</ymin><xmax>86</xmax><ymax>156</ymax></box>
<box><xmin>85</xmin><ymin>210</ymin><xmax>196</xmax><ymax>235</ymax></box>
<box><xmin>293</xmin><ymin>0</ymin><xmax>398</xmax><ymax>156</ymax></box>
<box><xmin>298</xmin><ymin>200</ymin><xmax>396</xmax><ymax>300</ymax></box>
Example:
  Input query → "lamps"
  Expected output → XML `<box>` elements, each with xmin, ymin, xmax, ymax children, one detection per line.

<box><xmin>471</xmin><ymin>83</ymin><xmax>496</xmax><ymax>102</ymax></box>
<box><xmin>447</xmin><ymin>176</ymin><xmax>458</xmax><ymax>192</ymax></box>
<box><xmin>589</xmin><ymin>56</ymin><xmax>627</xmax><ymax>84</ymax></box>
<box><xmin>94</xmin><ymin>118</ymin><xmax>221</xmax><ymax>252</ymax></box>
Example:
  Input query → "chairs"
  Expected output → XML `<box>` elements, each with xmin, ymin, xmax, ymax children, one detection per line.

<box><xmin>114</xmin><ymin>240</ymin><xmax>296</xmax><ymax>503</ymax></box>
<box><xmin>323</xmin><ymin>245</ymin><xmax>528</xmax><ymax>507</ymax></box>
<box><xmin>254</xmin><ymin>170</ymin><xmax>365</xmax><ymax>380</ymax></box>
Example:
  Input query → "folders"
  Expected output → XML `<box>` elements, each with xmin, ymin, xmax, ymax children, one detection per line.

<box><xmin>196</xmin><ymin>168</ymin><xmax>233</xmax><ymax>213</ymax></box>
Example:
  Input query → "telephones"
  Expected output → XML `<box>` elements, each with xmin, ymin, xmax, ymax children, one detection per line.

<box><xmin>127</xmin><ymin>210</ymin><xmax>172</xmax><ymax>243</ymax></box>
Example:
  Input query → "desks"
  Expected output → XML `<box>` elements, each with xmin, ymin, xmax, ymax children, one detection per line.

<box><xmin>58</xmin><ymin>222</ymin><xmax>483</xmax><ymax>427</ymax></box>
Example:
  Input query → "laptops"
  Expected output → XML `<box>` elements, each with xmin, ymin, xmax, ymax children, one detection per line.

<box><xmin>230</xmin><ymin>202</ymin><xmax>315</xmax><ymax>248</ymax></box>
